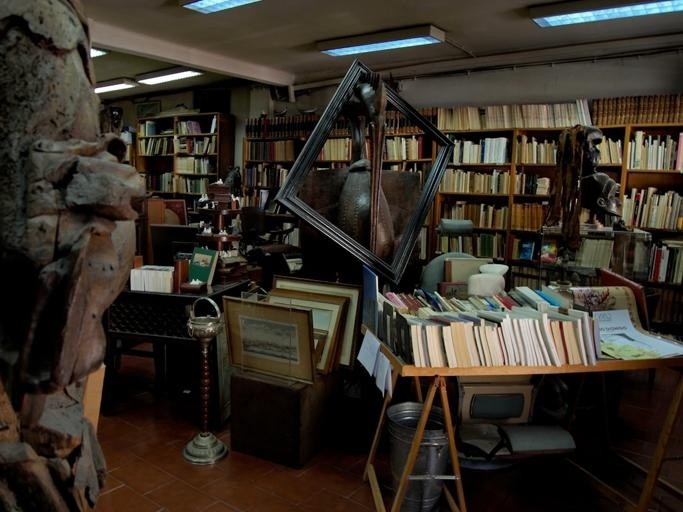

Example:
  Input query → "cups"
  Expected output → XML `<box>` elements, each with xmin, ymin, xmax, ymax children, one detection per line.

<box><xmin>174</xmin><ymin>255</ymin><xmax>190</xmax><ymax>294</ymax></box>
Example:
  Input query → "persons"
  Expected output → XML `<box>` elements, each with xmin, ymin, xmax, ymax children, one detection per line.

<box><xmin>107</xmin><ymin>138</ymin><xmax>128</xmax><ymax>164</ymax></box>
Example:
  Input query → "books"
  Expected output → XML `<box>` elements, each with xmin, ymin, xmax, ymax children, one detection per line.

<box><xmin>127</xmin><ymin>91</ymin><xmax>683</xmax><ymax>366</ymax></box>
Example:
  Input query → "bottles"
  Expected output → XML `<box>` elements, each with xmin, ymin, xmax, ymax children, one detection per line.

<box><xmin>550</xmin><ymin>278</ymin><xmax>575</xmax><ymax>309</ymax></box>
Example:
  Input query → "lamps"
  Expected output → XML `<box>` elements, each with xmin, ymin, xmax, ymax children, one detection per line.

<box><xmin>527</xmin><ymin>1</ymin><xmax>683</xmax><ymax>30</ymax></box>
<box><xmin>317</xmin><ymin>24</ymin><xmax>447</xmax><ymax>58</ymax></box>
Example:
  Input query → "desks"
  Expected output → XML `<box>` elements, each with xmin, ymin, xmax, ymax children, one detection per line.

<box><xmin>358</xmin><ymin>325</ymin><xmax>683</xmax><ymax>511</ymax></box>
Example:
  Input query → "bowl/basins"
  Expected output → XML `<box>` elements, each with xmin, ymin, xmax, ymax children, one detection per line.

<box><xmin>479</xmin><ymin>264</ymin><xmax>510</xmax><ymax>278</ymax></box>
<box><xmin>440</xmin><ymin>217</ymin><xmax>474</xmax><ymax>234</ymax></box>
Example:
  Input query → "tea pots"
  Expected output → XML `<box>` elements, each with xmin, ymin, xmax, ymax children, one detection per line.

<box><xmin>186</xmin><ymin>297</ymin><xmax>226</xmax><ymax>339</ymax></box>
